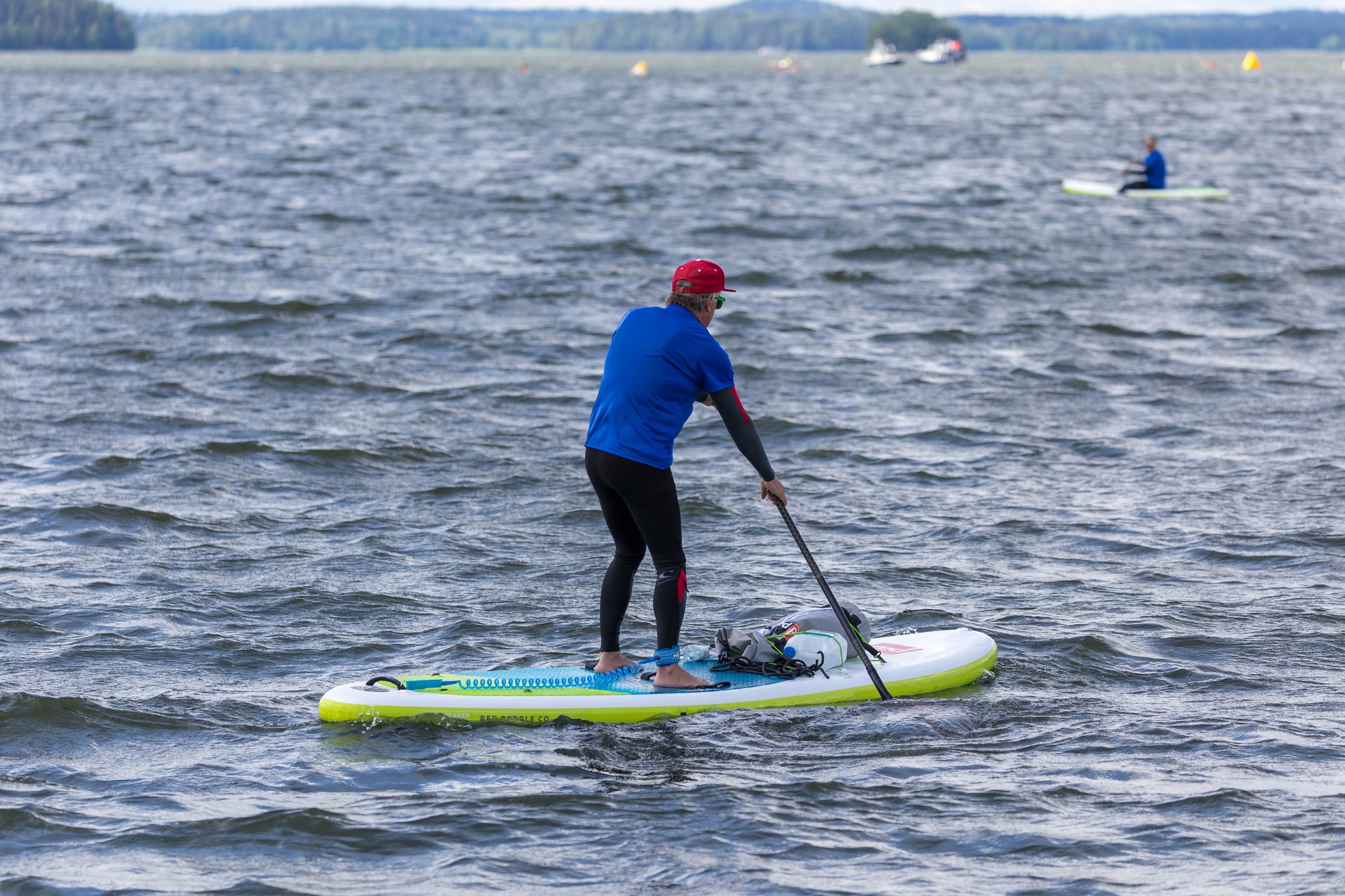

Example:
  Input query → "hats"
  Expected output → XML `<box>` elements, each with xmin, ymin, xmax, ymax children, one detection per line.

<box><xmin>671</xmin><ymin>259</ymin><xmax>737</xmax><ymax>293</ymax></box>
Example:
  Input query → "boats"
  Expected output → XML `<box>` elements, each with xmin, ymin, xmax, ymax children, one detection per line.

<box><xmin>863</xmin><ymin>51</ymin><xmax>903</xmax><ymax>69</ymax></box>
<box><xmin>916</xmin><ymin>45</ymin><xmax>970</xmax><ymax>65</ymax></box>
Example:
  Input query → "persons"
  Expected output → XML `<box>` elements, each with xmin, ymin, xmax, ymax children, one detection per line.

<box><xmin>1119</xmin><ymin>134</ymin><xmax>1164</xmax><ymax>195</ymax></box>
<box><xmin>583</xmin><ymin>259</ymin><xmax>789</xmax><ymax>689</ymax></box>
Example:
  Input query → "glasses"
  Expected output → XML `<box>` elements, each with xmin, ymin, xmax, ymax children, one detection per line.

<box><xmin>701</xmin><ymin>294</ymin><xmax>725</xmax><ymax>310</ymax></box>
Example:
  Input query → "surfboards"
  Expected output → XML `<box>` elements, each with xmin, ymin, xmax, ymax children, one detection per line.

<box><xmin>1058</xmin><ymin>176</ymin><xmax>1229</xmax><ymax>204</ymax></box>
<box><xmin>315</xmin><ymin>626</ymin><xmax>999</xmax><ymax>729</ymax></box>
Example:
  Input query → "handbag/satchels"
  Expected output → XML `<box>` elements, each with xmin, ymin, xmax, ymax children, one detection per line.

<box><xmin>757</xmin><ymin>598</ymin><xmax>870</xmax><ymax>658</ymax></box>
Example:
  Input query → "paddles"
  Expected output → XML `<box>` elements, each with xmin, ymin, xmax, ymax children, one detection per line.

<box><xmin>771</xmin><ymin>493</ymin><xmax>893</xmax><ymax>700</ymax></box>
